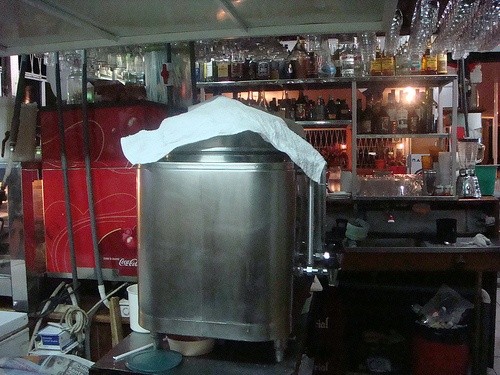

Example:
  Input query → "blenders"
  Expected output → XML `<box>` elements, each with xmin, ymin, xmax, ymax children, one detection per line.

<box><xmin>456</xmin><ymin>137</ymin><xmax>486</xmax><ymax>199</ymax></box>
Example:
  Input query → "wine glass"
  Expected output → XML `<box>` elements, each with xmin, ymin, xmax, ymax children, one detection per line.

<box><xmin>192</xmin><ymin>31</ymin><xmax>377</xmax><ymax>78</ymax></box>
<box><xmin>34</xmin><ymin>41</ymin><xmax>192</xmax><ymax>86</ymax></box>
<box><xmin>382</xmin><ymin>0</ymin><xmax>500</xmax><ymax>63</ymax></box>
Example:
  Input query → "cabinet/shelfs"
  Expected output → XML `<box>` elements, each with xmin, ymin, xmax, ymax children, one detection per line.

<box><xmin>198</xmin><ymin>73</ymin><xmax>458</xmax><ymax>199</ymax></box>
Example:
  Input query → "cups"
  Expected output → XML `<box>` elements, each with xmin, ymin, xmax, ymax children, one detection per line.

<box><xmin>328</xmin><ymin>167</ymin><xmax>341</xmax><ymax>193</ymax></box>
<box><xmin>420</xmin><ymin>155</ymin><xmax>433</xmax><ymax>169</ymax></box>
<box><xmin>430</xmin><ymin>146</ymin><xmax>442</xmax><ymax>162</ymax></box>
<box><xmin>375</xmin><ymin>160</ymin><xmax>384</xmax><ymax>170</ymax></box>
<box><xmin>438</xmin><ymin>137</ymin><xmax>448</xmax><ymax>152</ymax></box>
<box><xmin>424</xmin><ymin>170</ymin><xmax>438</xmax><ymax>196</ymax></box>
<box><xmin>436</xmin><ymin>218</ymin><xmax>457</xmax><ymax>244</ymax></box>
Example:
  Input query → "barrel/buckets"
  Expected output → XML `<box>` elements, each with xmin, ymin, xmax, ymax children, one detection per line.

<box><xmin>126</xmin><ymin>283</ymin><xmax>151</xmax><ymax>333</ymax></box>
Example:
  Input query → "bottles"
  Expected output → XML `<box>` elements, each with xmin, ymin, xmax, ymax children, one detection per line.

<box><xmin>356</xmin><ymin>88</ymin><xmax>438</xmax><ymax>134</ymax></box>
<box><xmin>293</xmin><ymin>40</ymin><xmax>311</xmax><ymax>78</ymax></box>
<box><xmin>420</xmin><ymin>306</ymin><xmax>446</xmax><ymax>327</ymax></box>
<box><xmin>233</xmin><ymin>91</ymin><xmax>352</xmax><ymax>121</ymax></box>
<box><xmin>67</xmin><ymin>66</ymin><xmax>83</xmax><ymax>104</ymax></box>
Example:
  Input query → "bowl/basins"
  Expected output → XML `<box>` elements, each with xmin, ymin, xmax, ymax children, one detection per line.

<box><xmin>166</xmin><ymin>334</ymin><xmax>215</xmax><ymax>356</ymax></box>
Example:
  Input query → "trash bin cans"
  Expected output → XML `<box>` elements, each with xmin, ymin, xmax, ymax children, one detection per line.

<box><xmin>412</xmin><ymin>317</ymin><xmax>471</xmax><ymax>375</ymax></box>
